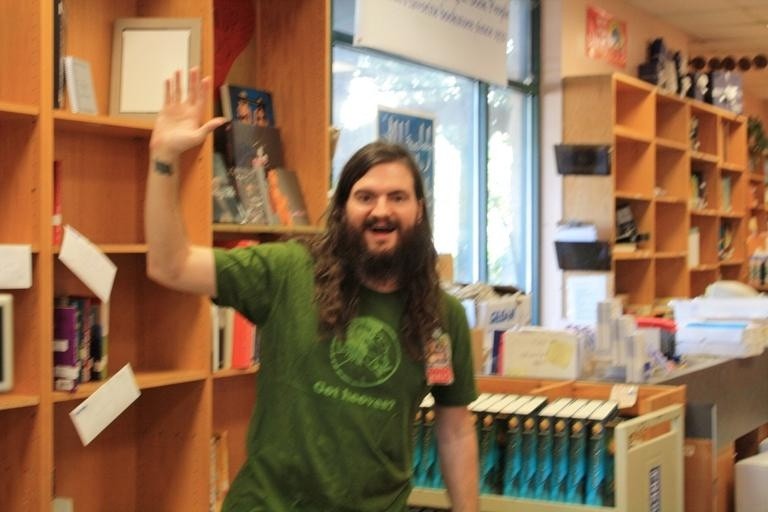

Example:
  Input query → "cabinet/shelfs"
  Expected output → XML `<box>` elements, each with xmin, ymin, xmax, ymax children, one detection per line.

<box><xmin>406</xmin><ymin>378</ymin><xmax>685</xmax><ymax>512</ymax></box>
<box><xmin>0</xmin><ymin>0</ymin><xmax>332</xmax><ymax>512</ymax></box>
<box><xmin>560</xmin><ymin>72</ymin><xmax>768</xmax><ymax>327</ymax></box>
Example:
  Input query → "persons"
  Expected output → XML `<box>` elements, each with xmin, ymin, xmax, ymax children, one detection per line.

<box><xmin>142</xmin><ymin>68</ymin><xmax>480</xmax><ymax>512</ymax></box>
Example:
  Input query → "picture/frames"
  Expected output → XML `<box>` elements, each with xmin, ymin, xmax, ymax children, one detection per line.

<box><xmin>108</xmin><ymin>17</ymin><xmax>203</xmax><ymax>129</ymax></box>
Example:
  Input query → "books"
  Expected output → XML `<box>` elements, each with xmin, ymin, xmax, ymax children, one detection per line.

<box><xmin>67</xmin><ymin>55</ymin><xmax>98</xmax><ymax>114</ymax></box>
<box><xmin>213</xmin><ymin>82</ymin><xmax>318</xmax><ymax>228</ymax></box>
<box><xmin>55</xmin><ymin>293</ymin><xmax>112</xmax><ymax>391</ymax></box>
<box><xmin>55</xmin><ymin>5</ymin><xmax>65</xmax><ymax>106</ymax></box>
<box><xmin>411</xmin><ymin>393</ymin><xmax>619</xmax><ymax>505</ymax></box>
<box><xmin>216</xmin><ymin>237</ymin><xmax>260</xmax><ymax>375</ymax></box>
